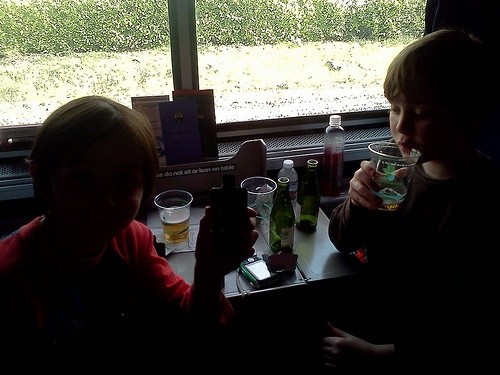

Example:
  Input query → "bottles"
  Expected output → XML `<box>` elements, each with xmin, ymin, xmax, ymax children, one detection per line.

<box><xmin>278</xmin><ymin>160</ymin><xmax>298</xmax><ymax>219</ymax></box>
<box><xmin>296</xmin><ymin>159</ymin><xmax>321</xmax><ymax>233</ymax></box>
<box><xmin>320</xmin><ymin>115</ymin><xmax>345</xmax><ymax>196</ymax></box>
<box><xmin>269</xmin><ymin>177</ymin><xmax>295</xmax><ymax>253</ymax></box>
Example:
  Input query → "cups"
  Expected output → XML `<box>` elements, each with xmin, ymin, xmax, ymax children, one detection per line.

<box><xmin>368</xmin><ymin>141</ymin><xmax>422</xmax><ymax>212</ymax></box>
<box><xmin>154</xmin><ymin>189</ymin><xmax>193</xmax><ymax>243</ymax></box>
<box><xmin>240</xmin><ymin>176</ymin><xmax>278</xmax><ymax>226</ymax></box>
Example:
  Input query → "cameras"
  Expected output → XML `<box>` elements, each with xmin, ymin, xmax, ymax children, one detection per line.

<box><xmin>237</xmin><ymin>255</ymin><xmax>281</xmax><ymax>289</ymax></box>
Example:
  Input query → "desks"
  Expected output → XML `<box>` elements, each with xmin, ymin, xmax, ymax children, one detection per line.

<box><xmin>146</xmin><ymin>203</ymin><xmax>380</xmax><ymax>316</ymax></box>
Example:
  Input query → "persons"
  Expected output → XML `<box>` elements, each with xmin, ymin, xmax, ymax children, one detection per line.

<box><xmin>320</xmin><ymin>28</ymin><xmax>500</xmax><ymax>375</ymax></box>
<box><xmin>0</xmin><ymin>97</ymin><xmax>259</xmax><ymax>375</ymax></box>
